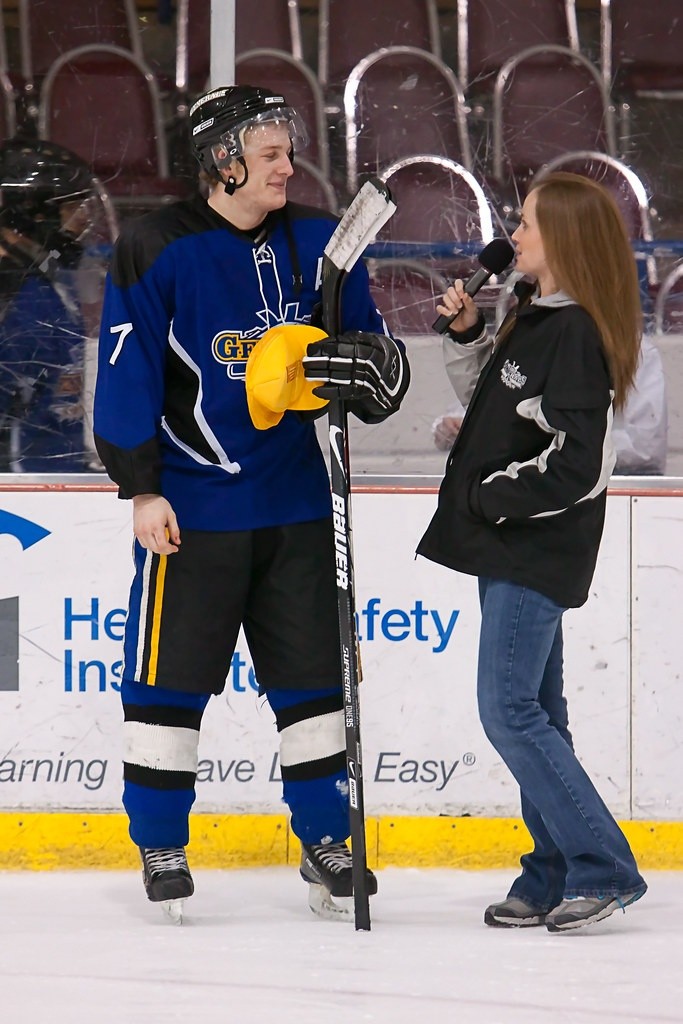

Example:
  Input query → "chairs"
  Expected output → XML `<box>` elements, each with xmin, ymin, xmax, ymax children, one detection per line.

<box><xmin>0</xmin><ymin>0</ymin><xmax>683</xmax><ymax>334</ymax></box>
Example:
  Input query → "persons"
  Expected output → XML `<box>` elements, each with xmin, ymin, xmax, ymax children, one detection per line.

<box><xmin>0</xmin><ymin>138</ymin><xmax>118</xmax><ymax>473</ymax></box>
<box><xmin>415</xmin><ymin>170</ymin><xmax>668</xmax><ymax>934</ymax></box>
<box><xmin>92</xmin><ymin>83</ymin><xmax>410</xmax><ymax>932</ymax></box>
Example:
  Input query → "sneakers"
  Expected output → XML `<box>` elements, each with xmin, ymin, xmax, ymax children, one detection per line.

<box><xmin>297</xmin><ymin>843</ymin><xmax>378</xmax><ymax>918</ymax></box>
<box><xmin>544</xmin><ymin>878</ymin><xmax>647</xmax><ymax>933</ymax></box>
<box><xmin>484</xmin><ymin>890</ymin><xmax>556</xmax><ymax>927</ymax></box>
<box><xmin>137</xmin><ymin>837</ymin><xmax>197</xmax><ymax>922</ymax></box>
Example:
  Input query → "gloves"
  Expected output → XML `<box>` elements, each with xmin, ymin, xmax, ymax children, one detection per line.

<box><xmin>297</xmin><ymin>331</ymin><xmax>414</xmax><ymax>423</ymax></box>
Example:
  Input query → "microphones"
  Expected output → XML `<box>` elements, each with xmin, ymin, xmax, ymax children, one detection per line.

<box><xmin>432</xmin><ymin>239</ymin><xmax>515</xmax><ymax>335</ymax></box>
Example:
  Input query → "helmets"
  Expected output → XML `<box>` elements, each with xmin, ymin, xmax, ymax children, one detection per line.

<box><xmin>186</xmin><ymin>87</ymin><xmax>295</xmax><ymax>175</ymax></box>
<box><xmin>0</xmin><ymin>140</ymin><xmax>97</xmax><ymax>270</ymax></box>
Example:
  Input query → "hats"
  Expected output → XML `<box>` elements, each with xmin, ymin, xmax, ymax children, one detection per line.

<box><xmin>246</xmin><ymin>322</ymin><xmax>331</xmax><ymax>430</ymax></box>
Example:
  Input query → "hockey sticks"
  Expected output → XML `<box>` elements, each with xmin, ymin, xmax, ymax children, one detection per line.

<box><xmin>315</xmin><ymin>173</ymin><xmax>398</xmax><ymax>931</ymax></box>
